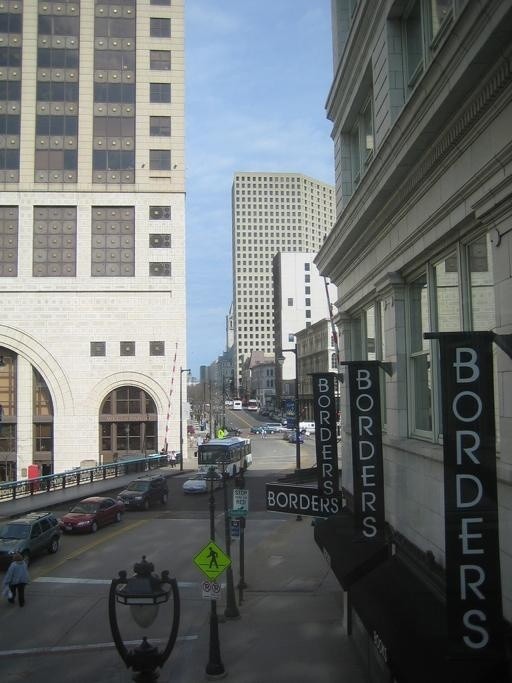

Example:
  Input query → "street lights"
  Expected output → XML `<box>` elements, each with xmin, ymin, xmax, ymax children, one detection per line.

<box><xmin>275</xmin><ymin>343</ymin><xmax>302</xmax><ymax>475</ymax></box>
<box><xmin>179</xmin><ymin>368</ymin><xmax>192</xmax><ymax>472</ymax></box>
<box><xmin>201</xmin><ymin>464</ymin><xmax>230</xmax><ymax>680</ymax></box>
<box><xmin>104</xmin><ymin>552</ymin><xmax>183</xmax><ymax>683</ymax></box>
<box><xmin>202</xmin><ymin>374</ymin><xmax>233</xmax><ymax>435</ymax></box>
<box><xmin>213</xmin><ymin>452</ymin><xmax>242</xmax><ymax>620</ymax></box>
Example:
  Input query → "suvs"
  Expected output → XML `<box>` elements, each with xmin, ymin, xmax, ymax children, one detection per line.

<box><xmin>114</xmin><ymin>473</ymin><xmax>170</xmax><ymax>510</ymax></box>
<box><xmin>0</xmin><ymin>509</ymin><xmax>63</xmax><ymax>569</ymax></box>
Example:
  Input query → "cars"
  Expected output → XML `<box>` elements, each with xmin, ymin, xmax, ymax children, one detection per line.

<box><xmin>57</xmin><ymin>494</ymin><xmax>127</xmax><ymax>535</ymax></box>
<box><xmin>179</xmin><ymin>471</ymin><xmax>225</xmax><ymax>495</ymax></box>
<box><xmin>250</xmin><ymin>420</ymin><xmax>316</xmax><ymax>444</ymax></box>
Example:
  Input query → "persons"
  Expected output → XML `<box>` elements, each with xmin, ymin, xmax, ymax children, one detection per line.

<box><xmin>169</xmin><ymin>451</ymin><xmax>177</xmax><ymax>468</ymax></box>
<box><xmin>3</xmin><ymin>554</ymin><xmax>32</xmax><ymax>607</ymax></box>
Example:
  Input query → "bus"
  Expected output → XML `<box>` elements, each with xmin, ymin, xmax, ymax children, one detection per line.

<box><xmin>193</xmin><ymin>436</ymin><xmax>255</xmax><ymax>481</ymax></box>
<box><xmin>233</xmin><ymin>400</ymin><xmax>243</xmax><ymax>410</ymax></box>
<box><xmin>246</xmin><ymin>398</ymin><xmax>259</xmax><ymax>411</ymax></box>
